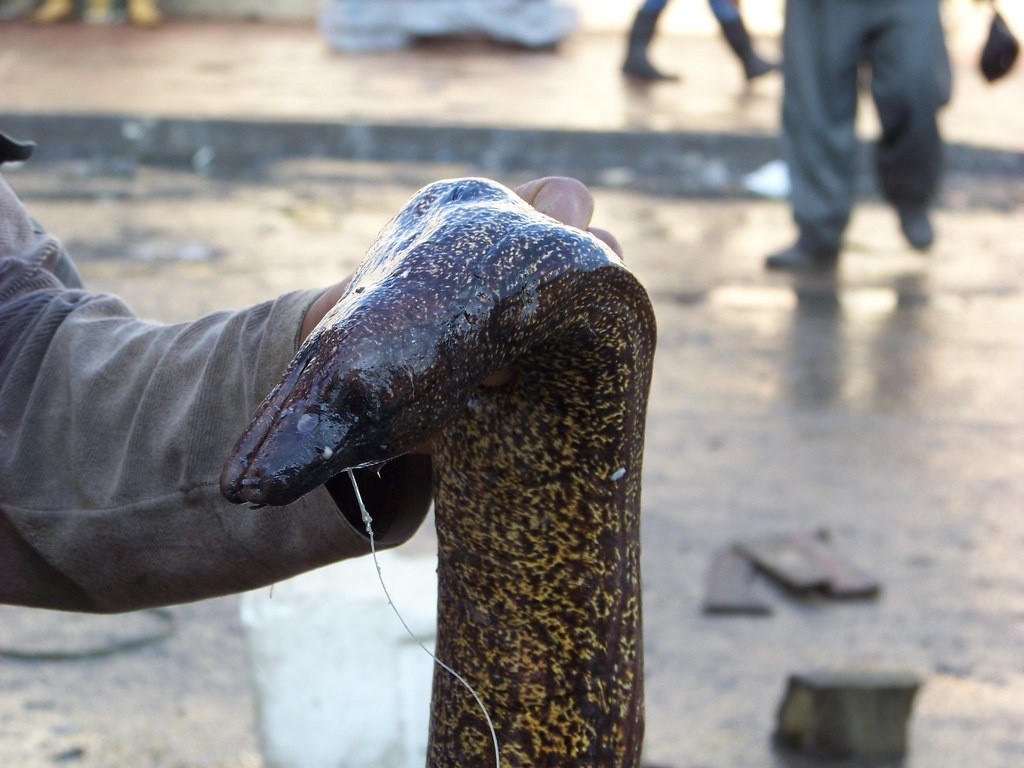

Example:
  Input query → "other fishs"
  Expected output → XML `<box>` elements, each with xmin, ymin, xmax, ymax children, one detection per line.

<box><xmin>220</xmin><ymin>174</ymin><xmax>658</xmax><ymax>768</ymax></box>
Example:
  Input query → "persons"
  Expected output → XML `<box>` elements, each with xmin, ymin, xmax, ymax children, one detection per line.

<box><xmin>620</xmin><ymin>0</ymin><xmax>779</xmax><ymax>84</ymax></box>
<box><xmin>767</xmin><ymin>0</ymin><xmax>951</xmax><ymax>268</ymax></box>
<box><xmin>0</xmin><ymin>121</ymin><xmax>623</xmax><ymax>610</ymax></box>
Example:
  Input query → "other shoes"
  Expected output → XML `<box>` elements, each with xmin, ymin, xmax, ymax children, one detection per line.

<box><xmin>766</xmin><ymin>231</ymin><xmax>840</xmax><ymax>270</ymax></box>
<box><xmin>899</xmin><ymin>203</ymin><xmax>934</xmax><ymax>247</ymax></box>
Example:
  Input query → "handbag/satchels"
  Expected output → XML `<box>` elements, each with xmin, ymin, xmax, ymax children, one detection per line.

<box><xmin>979</xmin><ymin>12</ymin><xmax>1020</xmax><ymax>84</ymax></box>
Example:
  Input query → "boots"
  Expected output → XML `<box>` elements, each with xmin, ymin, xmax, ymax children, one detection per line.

<box><xmin>722</xmin><ymin>15</ymin><xmax>777</xmax><ymax>77</ymax></box>
<box><xmin>622</xmin><ymin>10</ymin><xmax>664</xmax><ymax>79</ymax></box>
